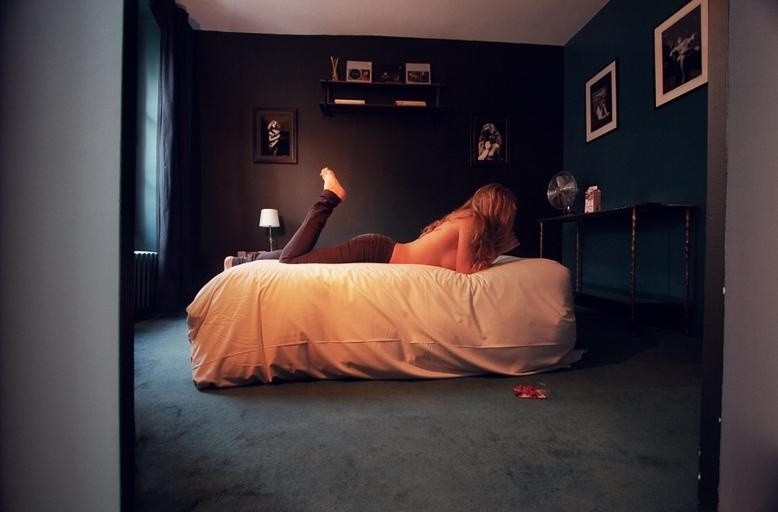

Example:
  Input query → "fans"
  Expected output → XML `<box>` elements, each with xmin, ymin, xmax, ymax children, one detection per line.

<box><xmin>547</xmin><ymin>171</ymin><xmax>578</xmax><ymax>216</ymax></box>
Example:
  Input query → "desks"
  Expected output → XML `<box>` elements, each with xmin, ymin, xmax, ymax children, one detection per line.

<box><xmin>537</xmin><ymin>201</ymin><xmax>702</xmax><ymax>323</ymax></box>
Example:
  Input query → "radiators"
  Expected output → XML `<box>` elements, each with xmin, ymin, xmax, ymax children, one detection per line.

<box><xmin>134</xmin><ymin>250</ymin><xmax>158</xmax><ymax>309</ymax></box>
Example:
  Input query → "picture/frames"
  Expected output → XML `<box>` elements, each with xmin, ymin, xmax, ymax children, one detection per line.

<box><xmin>405</xmin><ymin>63</ymin><xmax>431</xmax><ymax>85</ymax></box>
<box><xmin>346</xmin><ymin>60</ymin><xmax>372</xmax><ymax>83</ymax></box>
<box><xmin>654</xmin><ymin>0</ymin><xmax>709</xmax><ymax>107</ymax></box>
<box><xmin>250</xmin><ymin>105</ymin><xmax>298</xmax><ymax>164</ymax></box>
<box><xmin>585</xmin><ymin>60</ymin><xmax>617</xmax><ymax>142</ymax></box>
<box><xmin>469</xmin><ymin>105</ymin><xmax>511</xmax><ymax>168</ymax></box>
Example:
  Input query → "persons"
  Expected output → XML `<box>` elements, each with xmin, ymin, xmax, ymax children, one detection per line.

<box><xmin>476</xmin><ymin>123</ymin><xmax>504</xmax><ymax>161</ymax></box>
<box><xmin>594</xmin><ymin>94</ymin><xmax>611</xmax><ymax>121</ymax></box>
<box><xmin>267</xmin><ymin>120</ymin><xmax>282</xmax><ymax>156</ymax></box>
<box><xmin>222</xmin><ymin>164</ymin><xmax>523</xmax><ymax>275</ymax></box>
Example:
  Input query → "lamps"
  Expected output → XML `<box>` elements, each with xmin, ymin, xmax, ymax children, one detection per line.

<box><xmin>259</xmin><ymin>208</ymin><xmax>280</xmax><ymax>252</ymax></box>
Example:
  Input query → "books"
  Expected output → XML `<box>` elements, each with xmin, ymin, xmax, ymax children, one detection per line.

<box><xmin>394</xmin><ymin>100</ymin><xmax>427</xmax><ymax>107</ymax></box>
<box><xmin>333</xmin><ymin>99</ymin><xmax>366</xmax><ymax>105</ymax></box>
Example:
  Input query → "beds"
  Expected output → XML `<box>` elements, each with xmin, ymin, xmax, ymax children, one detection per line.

<box><xmin>185</xmin><ymin>248</ymin><xmax>585</xmax><ymax>388</ymax></box>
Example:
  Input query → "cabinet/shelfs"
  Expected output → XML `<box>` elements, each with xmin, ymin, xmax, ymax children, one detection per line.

<box><xmin>318</xmin><ymin>78</ymin><xmax>448</xmax><ymax>115</ymax></box>
